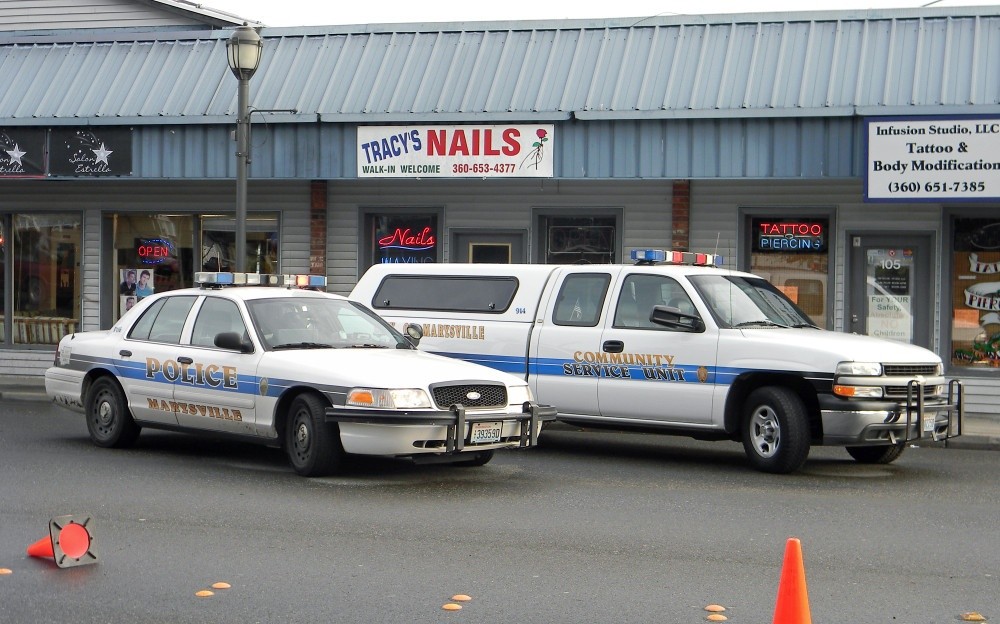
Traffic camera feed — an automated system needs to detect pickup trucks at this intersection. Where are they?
[334,247,968,475]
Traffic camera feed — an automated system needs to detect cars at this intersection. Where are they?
[44,272,558,480]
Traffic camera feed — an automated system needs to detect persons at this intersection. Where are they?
[122,296,134,317]
[137,270,153,295]
[120,270,137,296]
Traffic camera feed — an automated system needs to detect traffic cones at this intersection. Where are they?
[27,511,98,568]
[768,537,814,624]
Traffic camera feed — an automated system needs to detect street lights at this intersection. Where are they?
[221,22,268,275]
